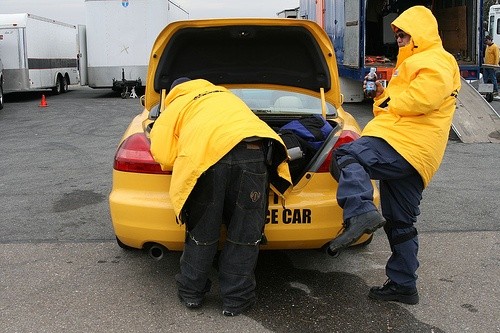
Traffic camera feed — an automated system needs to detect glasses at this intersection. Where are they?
[394,33,408,40]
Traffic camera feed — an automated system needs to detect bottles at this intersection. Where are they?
[364,67,377,99]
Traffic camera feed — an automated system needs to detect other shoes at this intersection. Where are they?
[222,309,236,316]
[182,293,199,307]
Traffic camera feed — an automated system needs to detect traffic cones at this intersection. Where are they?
[38,95,49,107]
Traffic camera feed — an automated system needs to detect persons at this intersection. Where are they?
[150,78,292,316]
[483,36,500,97]
[329,6,462,306]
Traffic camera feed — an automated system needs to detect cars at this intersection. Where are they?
[108,18,381,261]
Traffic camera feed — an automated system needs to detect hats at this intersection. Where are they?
[485,35,492,40]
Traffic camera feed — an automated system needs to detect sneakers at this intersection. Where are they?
[330,210,386,251]
[369,277,419,305]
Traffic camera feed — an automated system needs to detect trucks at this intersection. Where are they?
[0,13,79,95]
[277,0,481,103]
